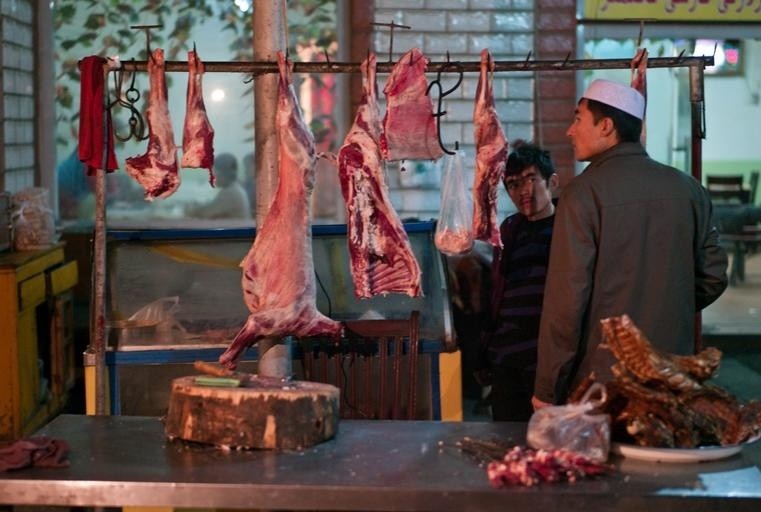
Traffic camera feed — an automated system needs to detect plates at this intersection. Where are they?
[605,432,752,463]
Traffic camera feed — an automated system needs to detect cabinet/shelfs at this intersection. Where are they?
[2,239,80,445]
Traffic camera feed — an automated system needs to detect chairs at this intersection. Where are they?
[299,308,421,420]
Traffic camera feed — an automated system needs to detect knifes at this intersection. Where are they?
[193,376,264,389]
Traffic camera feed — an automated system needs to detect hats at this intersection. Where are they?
[579,78,646,122]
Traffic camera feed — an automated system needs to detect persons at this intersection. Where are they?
[237,152,256,221]
[529,75,731,417]
[457,137,563,423]
[186,151,251,221]
[56,114,136,219]
